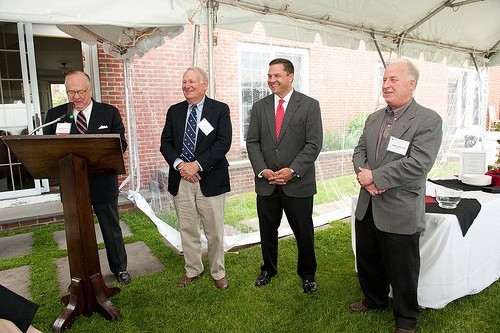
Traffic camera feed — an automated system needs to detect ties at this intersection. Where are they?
[275,100,284,143]
[182,104,197,162]
[75,110,87,135]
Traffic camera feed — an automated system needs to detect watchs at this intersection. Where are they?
[290,168,297,177]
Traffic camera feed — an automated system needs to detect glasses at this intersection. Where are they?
[67,85,90,95]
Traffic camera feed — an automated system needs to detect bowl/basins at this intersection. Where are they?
[435,186,463,209]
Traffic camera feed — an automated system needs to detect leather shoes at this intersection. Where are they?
[396,328,414,333]
[302,279,317,296]
[177,270,204,288]
[349,300,386,312]
[115,271,132,285]
[214,277,227,290]
[255,269,271,286]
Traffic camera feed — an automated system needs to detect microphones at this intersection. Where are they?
[28,115,66,134]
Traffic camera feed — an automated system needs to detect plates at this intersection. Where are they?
[461,174,492,187]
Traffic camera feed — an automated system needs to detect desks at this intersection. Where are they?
[351,176,500,309]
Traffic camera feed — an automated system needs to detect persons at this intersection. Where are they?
[246,58,323,295]
[348,60,443,333]
[43,70,131,294]
[160,67,232,289]
